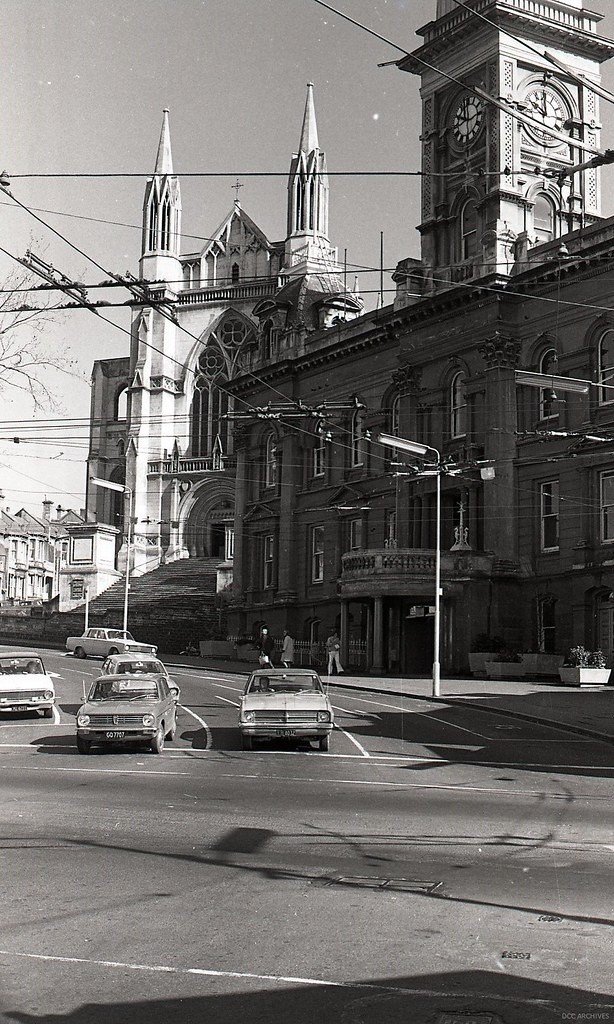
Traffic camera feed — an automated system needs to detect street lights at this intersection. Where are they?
[89,475,132,641]
[376,432,441,697]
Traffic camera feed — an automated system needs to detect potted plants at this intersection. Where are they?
[199,625,235,658]
[484,649,526,680]
[558,646,612,688]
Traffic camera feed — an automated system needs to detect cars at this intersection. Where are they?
[74,674,177,754]
[65,628,159,658]
[239,668,334,752]
[98,653,182,704]
[0,651,57,717]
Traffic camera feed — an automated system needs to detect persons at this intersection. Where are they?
[326,631,345,676]
[281,630,295,669]
[256,628,275,669]
[256,677,275,694]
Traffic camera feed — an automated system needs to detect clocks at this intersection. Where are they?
[443,85,487,153]
[520,81,573,148]
